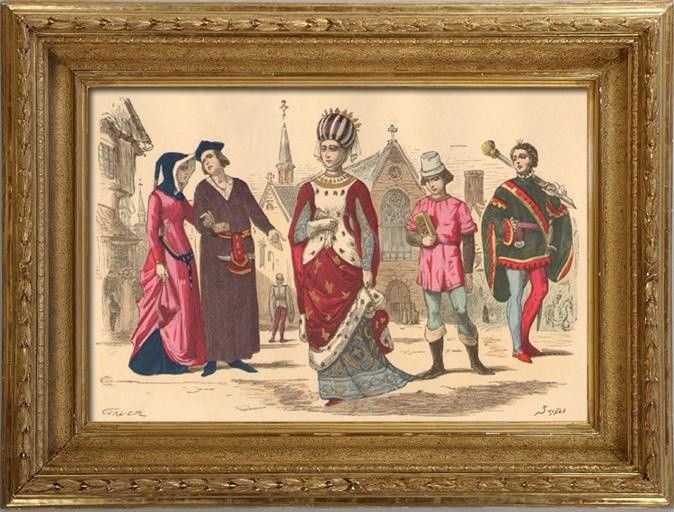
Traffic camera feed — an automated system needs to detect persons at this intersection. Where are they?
[107,294,120,332]
[126,152,214,377]
[403,150,494,379]
[481,138,575,363]
[193,140,286,376]
[287,108,417,406]
[266,272,294,341]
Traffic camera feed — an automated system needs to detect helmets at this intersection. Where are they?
[275,273,284,285]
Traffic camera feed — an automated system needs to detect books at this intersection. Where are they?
[413,210,434,237]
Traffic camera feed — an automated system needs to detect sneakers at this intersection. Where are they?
[523,344,542,357]
[269,339,288,343]
[228,360,258,373]
[200,361,217,377]
[512,350,532,364]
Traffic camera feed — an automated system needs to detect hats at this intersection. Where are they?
[155,152,196,198]
[195,140,224,161]
[316,113,357,151]
[420,150,446,178]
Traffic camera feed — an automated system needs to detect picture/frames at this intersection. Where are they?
[0,0,673,509]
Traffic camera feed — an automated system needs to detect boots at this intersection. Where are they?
[421,324,447,380]
[458,326,490,374]
